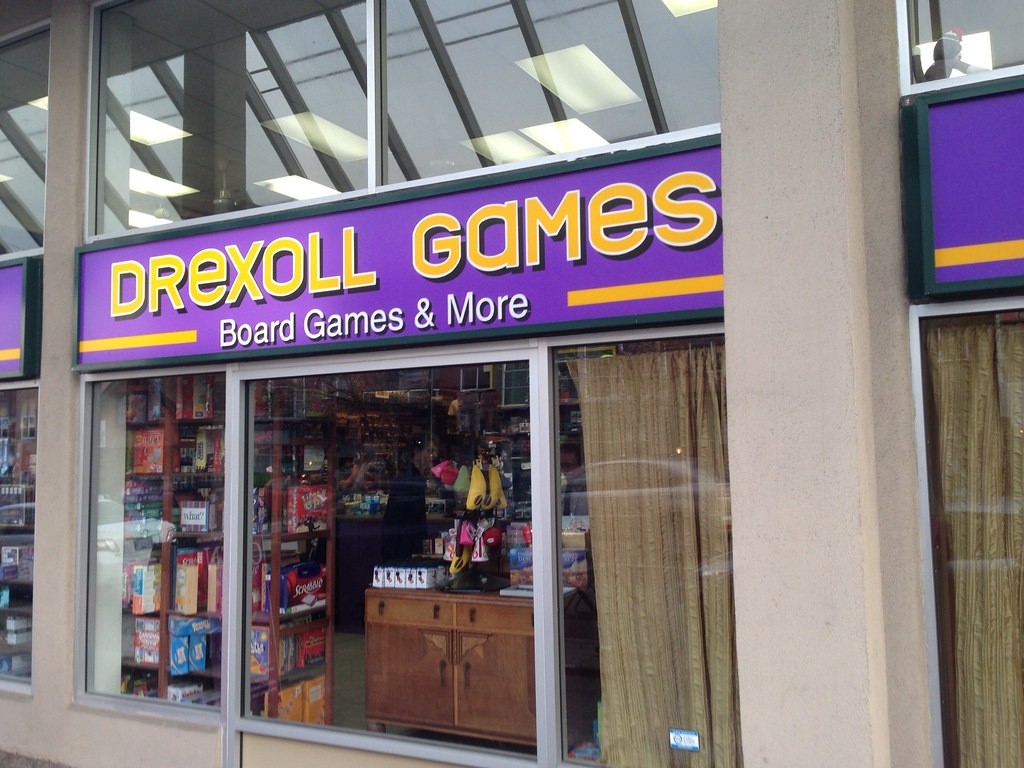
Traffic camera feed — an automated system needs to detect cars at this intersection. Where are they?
[518,458,732,530]
[0,502,177,595]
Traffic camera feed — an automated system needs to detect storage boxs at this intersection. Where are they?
[0,376,591,725]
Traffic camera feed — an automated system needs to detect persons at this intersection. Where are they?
[383,432,440,567]
[560,442,587,515]
[335,462,357,488]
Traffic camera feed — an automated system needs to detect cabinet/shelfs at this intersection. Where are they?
[0,373,340,726]
[364,588,583,746]
